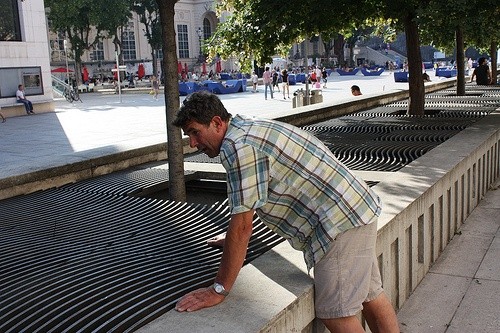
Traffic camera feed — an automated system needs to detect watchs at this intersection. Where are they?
[214,282,229,296]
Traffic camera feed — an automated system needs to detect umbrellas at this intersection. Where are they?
[83,66,89,82]
[178,62,188,74]
[114,65,117,79]
[202,62,207,74]
[217,60,221,73]
[138,64,145,79]
[51,67,74,78]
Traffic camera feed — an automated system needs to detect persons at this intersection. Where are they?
[468,57,492,85]
[124,72,133,84]
[403,61,407,71]
[450,59,454,67]
[16,85,34,114]
[385,61,393,71]
[289,65,305,73]
[351,85,362,96]
[310,66,327,88]
[431,56,434,64]
[181,69,221,80]
[251,67,290,100]
[172,90,401,333]
[384,46,388,53]
[151,75,159,101]
[90,74,102,85]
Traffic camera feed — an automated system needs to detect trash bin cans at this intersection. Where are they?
[311,90,323,104]
[293,89,310,108]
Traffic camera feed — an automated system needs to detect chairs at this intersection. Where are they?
[277,73,305,85]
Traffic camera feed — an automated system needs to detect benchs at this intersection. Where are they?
[394,72,409,82]
[425,62,434,68]
[436,67,458,77]
[179,79,242,96]
[337,64,385,76]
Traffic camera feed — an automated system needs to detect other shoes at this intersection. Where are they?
[27,111,35,115]
